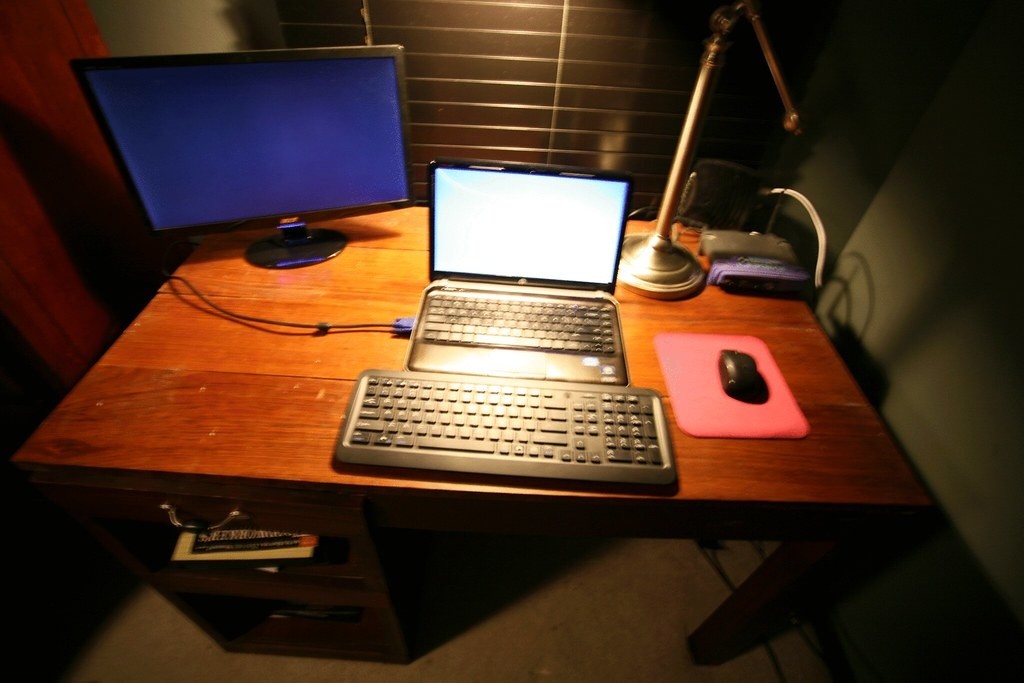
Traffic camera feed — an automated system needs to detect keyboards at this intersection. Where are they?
[332,371,678,495]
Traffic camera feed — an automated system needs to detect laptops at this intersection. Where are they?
[404,157,636,396]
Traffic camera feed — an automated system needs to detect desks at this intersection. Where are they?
[8,205,938,665]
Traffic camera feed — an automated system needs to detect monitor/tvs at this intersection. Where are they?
[70,44,416,268]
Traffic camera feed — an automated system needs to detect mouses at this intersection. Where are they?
[717,350,761,402]
